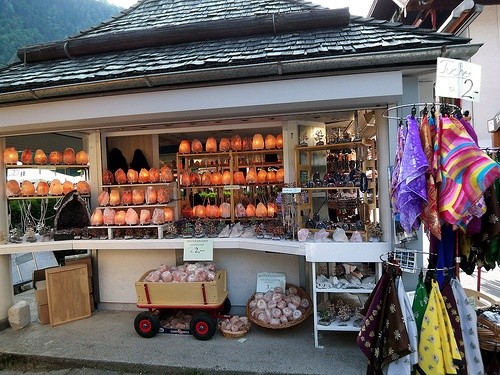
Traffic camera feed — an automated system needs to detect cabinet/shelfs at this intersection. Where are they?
[176,149,285,223]
[311,262,382,348]
[88,181,178,239]
[293,139,377,242]
[5,164,90,200]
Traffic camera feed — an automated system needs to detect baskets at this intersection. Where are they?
[222,329,247,339]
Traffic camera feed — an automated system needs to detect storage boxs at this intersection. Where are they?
[31,258,95,325]
[134,269,226,305]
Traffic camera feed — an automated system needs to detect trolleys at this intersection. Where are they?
[134,269,231,341]
